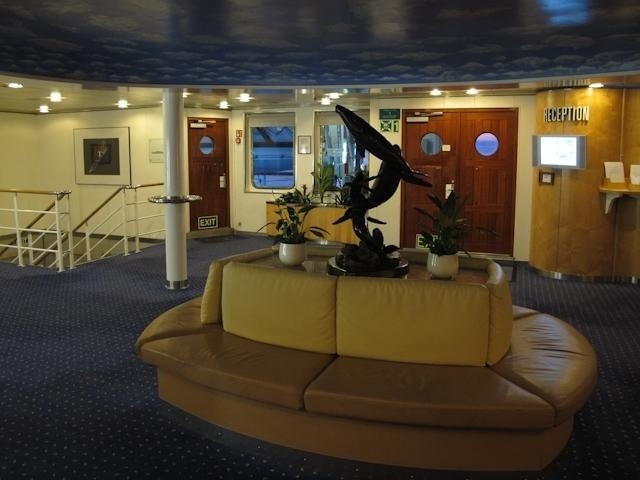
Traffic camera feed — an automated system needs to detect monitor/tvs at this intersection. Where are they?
[531,135,587,173]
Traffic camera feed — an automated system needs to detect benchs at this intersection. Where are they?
[134,244,598,472]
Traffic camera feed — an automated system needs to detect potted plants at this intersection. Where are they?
[411,190,503,278]
[256,184,331,266]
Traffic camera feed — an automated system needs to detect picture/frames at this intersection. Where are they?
[73,126,131,186]
[298,136,311,154]
[539,171,554,185]
